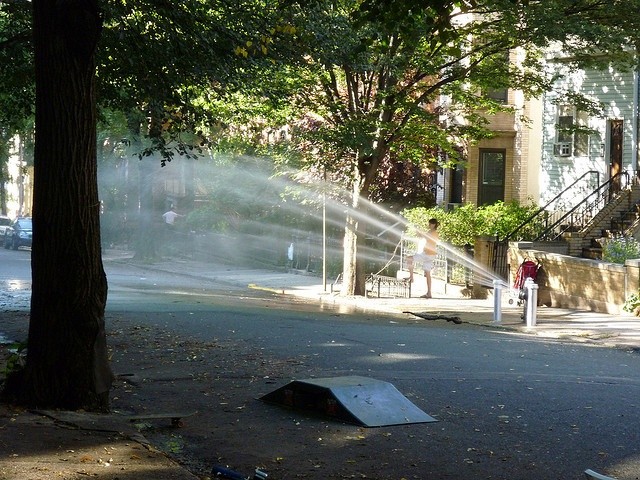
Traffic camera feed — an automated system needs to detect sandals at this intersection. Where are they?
[404,277,413,282]
[420,294,433,298]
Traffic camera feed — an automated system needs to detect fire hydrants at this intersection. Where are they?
[518,277,539,327]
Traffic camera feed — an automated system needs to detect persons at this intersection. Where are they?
[161,205,187,229]
[406,218,440,298]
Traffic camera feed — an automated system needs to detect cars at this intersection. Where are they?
[5,215,33,251]
[0,215,12,246]
